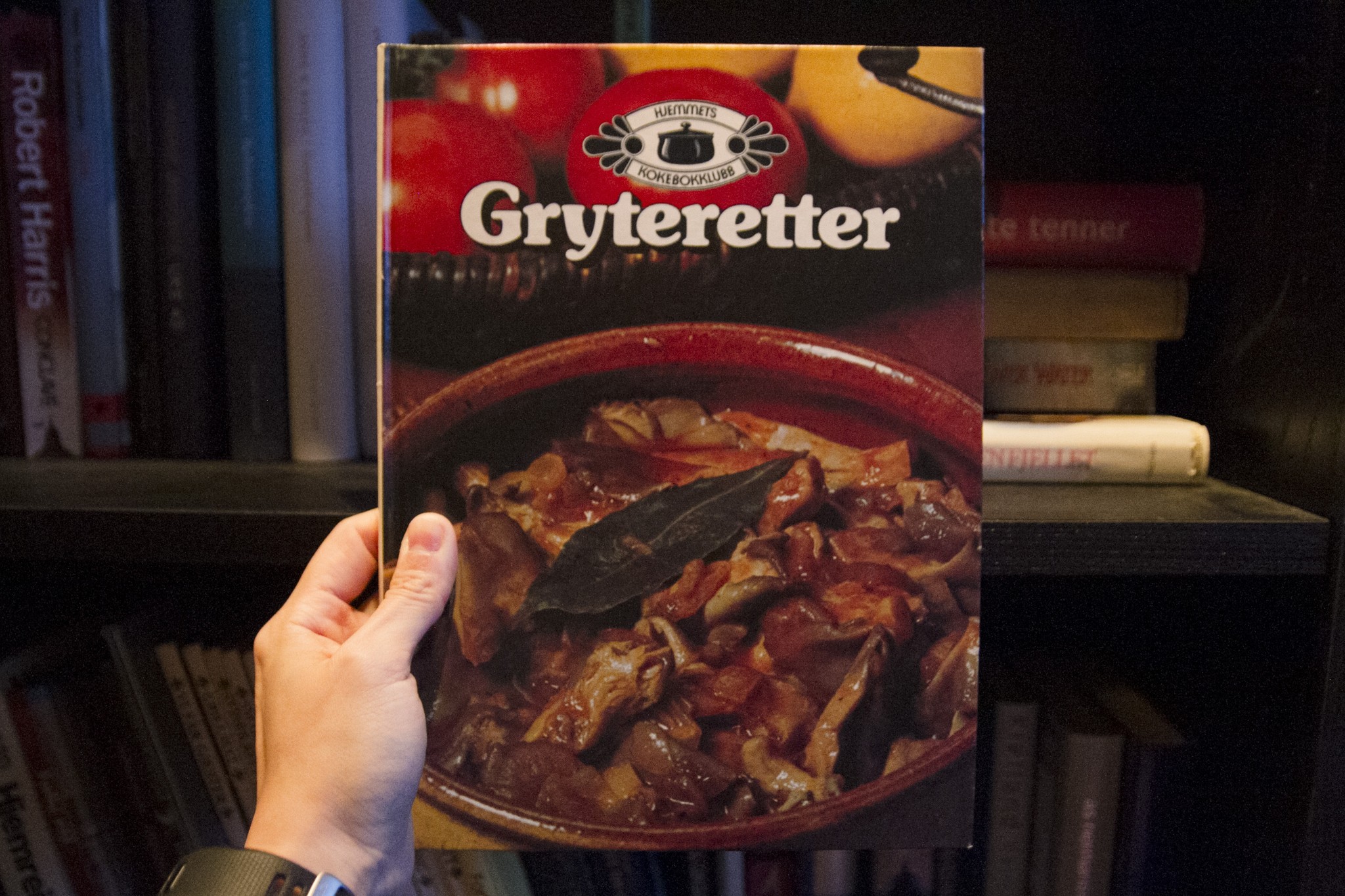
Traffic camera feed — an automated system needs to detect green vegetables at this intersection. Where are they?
[509,451,807,630]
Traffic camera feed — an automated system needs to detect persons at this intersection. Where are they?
[157,508,459,896]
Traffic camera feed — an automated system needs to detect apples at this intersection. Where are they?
[380,50,811,256]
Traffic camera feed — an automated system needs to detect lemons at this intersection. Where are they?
[587,48,986,176]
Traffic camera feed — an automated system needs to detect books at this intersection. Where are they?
[379,43,981,853]
[0,0,1219,896]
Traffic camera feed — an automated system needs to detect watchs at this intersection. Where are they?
[159,847,356,896]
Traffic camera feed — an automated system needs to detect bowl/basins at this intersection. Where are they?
[379,324,977,856]
